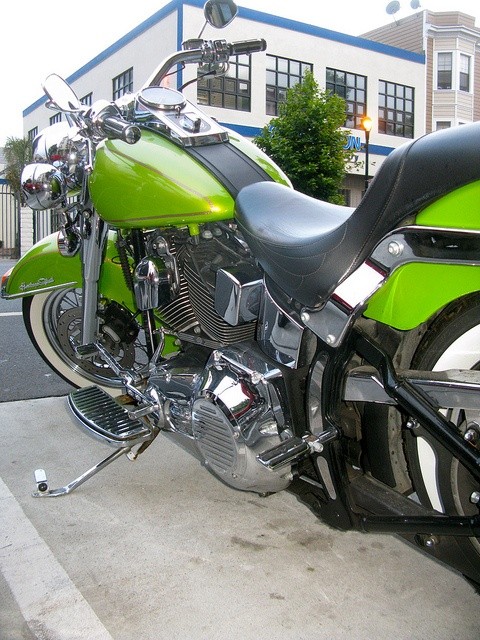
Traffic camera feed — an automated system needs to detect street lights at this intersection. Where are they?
[363,119,373,194]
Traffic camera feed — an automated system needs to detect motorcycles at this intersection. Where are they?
[0,0,480,594]
